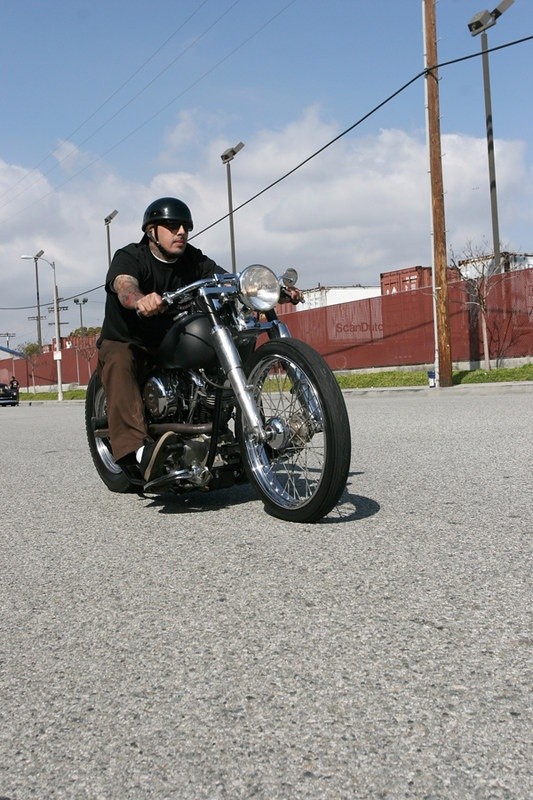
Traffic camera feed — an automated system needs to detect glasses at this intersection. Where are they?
[155,219,193,232]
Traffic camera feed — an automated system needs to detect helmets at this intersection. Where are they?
[141,197,194,231]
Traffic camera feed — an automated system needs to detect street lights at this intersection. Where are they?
[73,296,88,329]
[21,256,63,402]
[104,209,118,265]
[466,0,518,274]
[35,250,47,348]
[221,141,245,274]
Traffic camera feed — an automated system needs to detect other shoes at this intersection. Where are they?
[264,443,280,460]
[138,431,176,483]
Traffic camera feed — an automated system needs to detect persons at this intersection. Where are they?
[10,376,20,406]
[95,198,305,484]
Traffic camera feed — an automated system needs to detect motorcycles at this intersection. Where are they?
[83,265,354,524]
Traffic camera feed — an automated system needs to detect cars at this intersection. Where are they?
[0,382,19,407]
